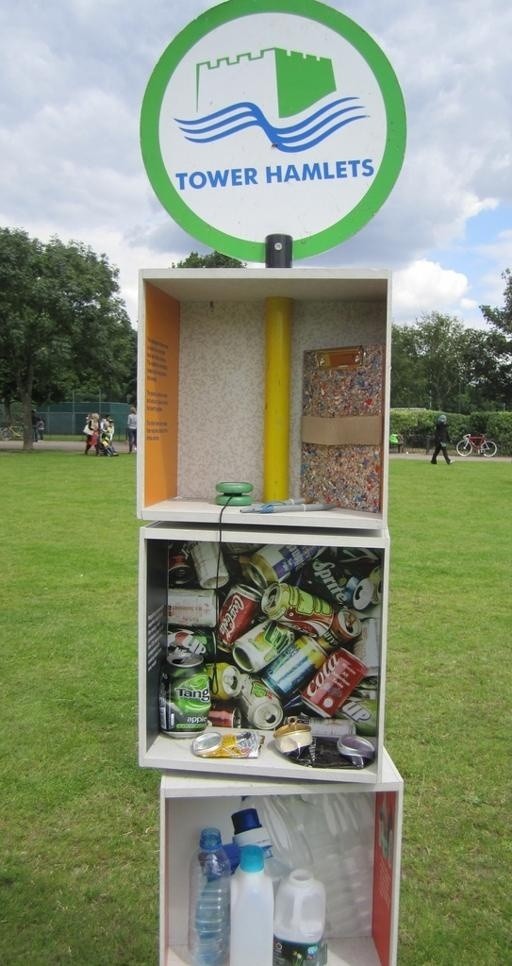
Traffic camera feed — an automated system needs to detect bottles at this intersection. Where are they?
[184,827,231,966]
[227,846,272,966]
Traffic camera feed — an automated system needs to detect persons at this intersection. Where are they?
[37,414,44,440]
[83,412,119,456]
[126,406,137,453]
[31,407,38,442]
[430,414,456,464]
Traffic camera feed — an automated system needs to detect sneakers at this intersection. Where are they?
[449,460,455,464]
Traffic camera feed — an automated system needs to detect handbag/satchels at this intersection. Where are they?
[83,425,95,435]
[440,442,446,447]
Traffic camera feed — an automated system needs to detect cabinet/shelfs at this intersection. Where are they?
[136,267,404,966]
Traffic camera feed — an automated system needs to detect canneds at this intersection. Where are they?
[272,724,375,768]
[191,723,264,758]
[159,543,383,736]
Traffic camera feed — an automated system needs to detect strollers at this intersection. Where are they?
[99,429,114,456]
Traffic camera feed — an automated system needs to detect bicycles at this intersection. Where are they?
[456,434,497,457]
[1,424,23,441]
